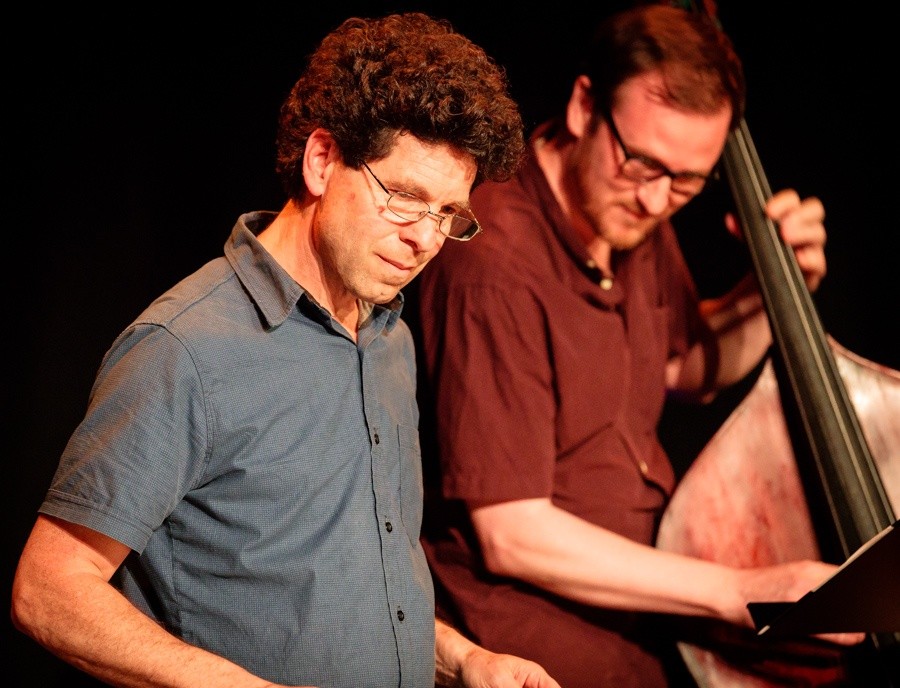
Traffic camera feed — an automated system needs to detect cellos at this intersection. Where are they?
[655,118,900,688]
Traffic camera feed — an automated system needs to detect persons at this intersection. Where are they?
[412,0,866,688]
[13,13,561,688]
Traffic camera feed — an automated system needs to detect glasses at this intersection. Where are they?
[595,87,720,197]
[360,159,484,241]
